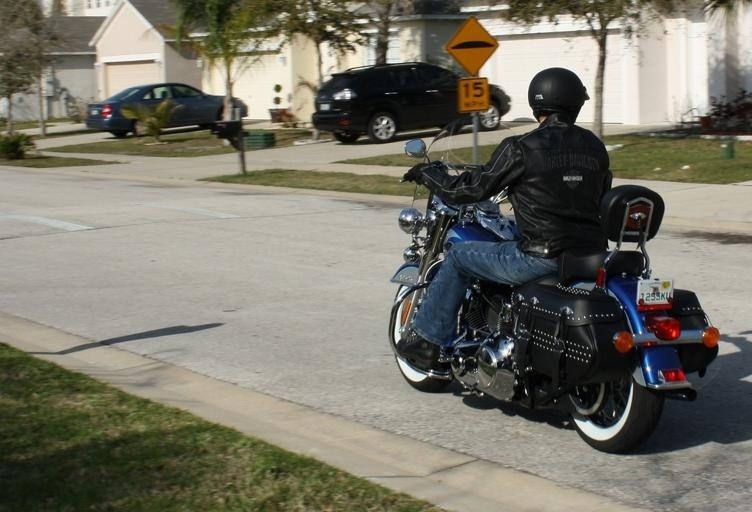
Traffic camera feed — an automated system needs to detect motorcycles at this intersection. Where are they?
[378,133,721,459]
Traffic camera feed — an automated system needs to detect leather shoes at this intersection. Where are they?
[396,336,440,369]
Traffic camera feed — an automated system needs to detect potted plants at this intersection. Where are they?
[269,83,289,122]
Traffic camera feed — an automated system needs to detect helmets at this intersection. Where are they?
[529,69,589,116]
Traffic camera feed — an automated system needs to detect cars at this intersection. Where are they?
[82,80,251,140]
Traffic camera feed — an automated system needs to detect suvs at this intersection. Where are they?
[310,59,513,145]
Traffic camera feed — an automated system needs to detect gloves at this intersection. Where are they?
[408,163,428,185]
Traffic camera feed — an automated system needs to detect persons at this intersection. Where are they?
[396,67,614,369]
